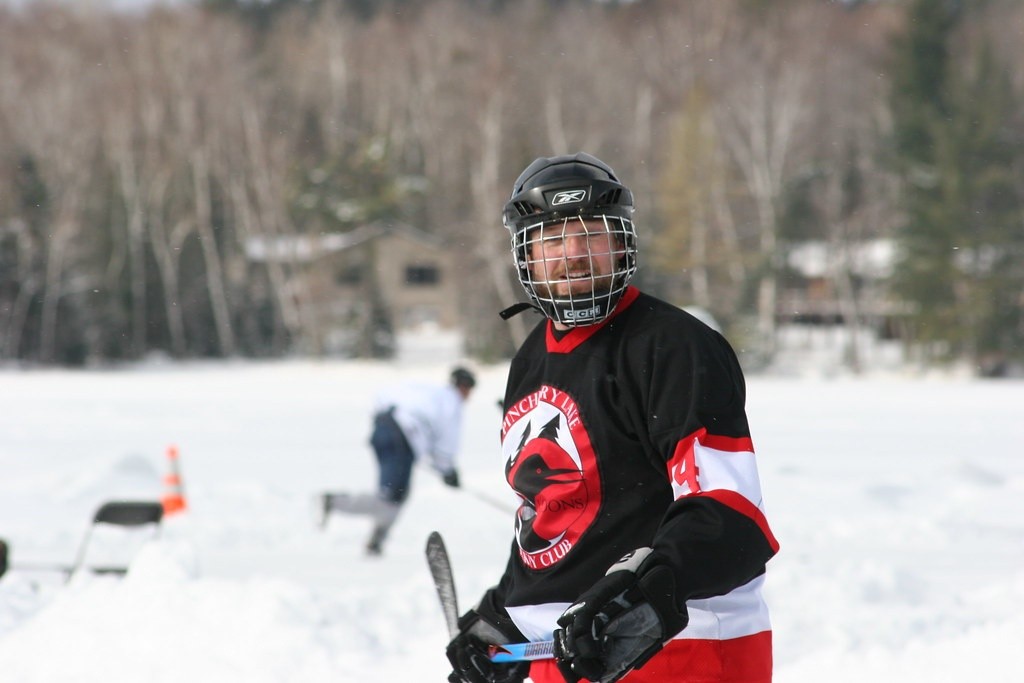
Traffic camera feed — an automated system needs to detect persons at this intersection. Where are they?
[447,154,780,683]
[318,367,475,558]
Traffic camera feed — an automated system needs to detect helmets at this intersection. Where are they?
[502,151,638,328]
[452,369,476,389]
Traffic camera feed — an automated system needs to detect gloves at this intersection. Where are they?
[446,588,534,683]
[552,546,689,683]
[446,472,458,487]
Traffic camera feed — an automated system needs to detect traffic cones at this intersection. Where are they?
[156,447,187,520]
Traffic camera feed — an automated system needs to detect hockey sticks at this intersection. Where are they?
[425,530,556,663]
